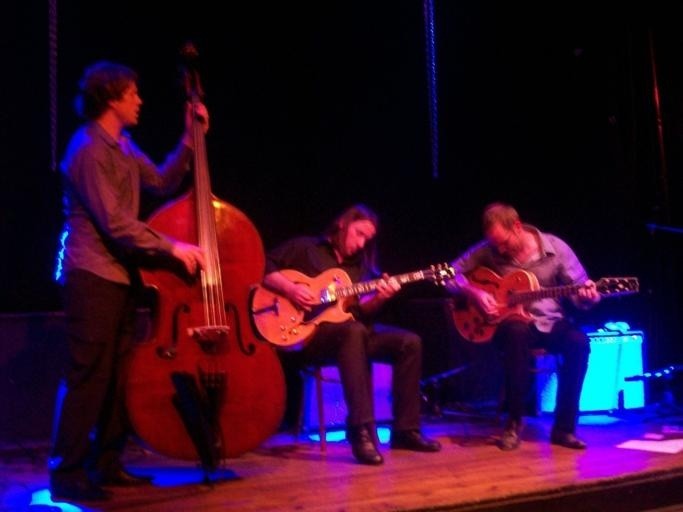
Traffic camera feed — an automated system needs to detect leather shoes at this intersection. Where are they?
[502,418,519,450]
[390,430,441,450]
[553,428,586,448]
[352,425,382,463]
[50,470,112,501]
[98,468,147,487]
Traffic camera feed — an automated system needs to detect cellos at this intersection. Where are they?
[126,41,285,458]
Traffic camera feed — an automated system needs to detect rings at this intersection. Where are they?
[189,259,196,265]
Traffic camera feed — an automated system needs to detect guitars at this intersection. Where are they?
[447,265,640,343]
[252,261,456,347]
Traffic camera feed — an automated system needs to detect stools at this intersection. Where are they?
[490,346,548,438]
[292,354,372,450]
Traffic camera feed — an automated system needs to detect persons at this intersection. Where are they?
[443,202,601,453]
[46,59,209,502]
[259,202,440,462]
